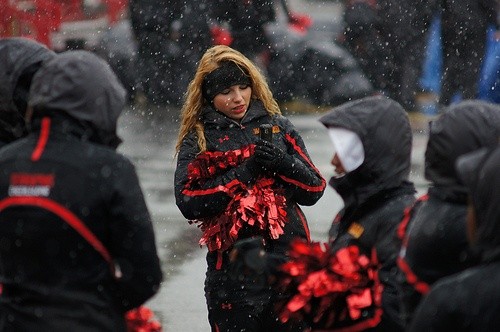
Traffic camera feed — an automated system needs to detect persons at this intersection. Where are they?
[0,34,59,150]
[0,48,163,331]
[396,100,500,332]
[409,148,500,332]
[317,94,419,331]
[173,44,326,332]
[123,1,500,121]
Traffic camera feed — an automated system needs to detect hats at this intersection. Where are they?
[329,124,365,171]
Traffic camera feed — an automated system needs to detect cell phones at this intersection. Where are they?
[260,124,273,144]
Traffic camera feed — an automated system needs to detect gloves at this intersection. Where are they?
[254,140,294,175]
[245,153,267,177]
